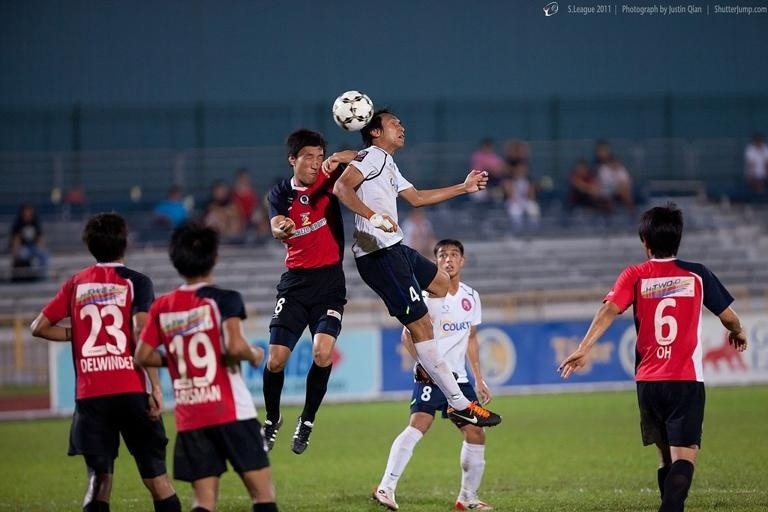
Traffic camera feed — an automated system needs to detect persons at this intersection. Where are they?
[29,211,184,512]
[8,203,50,282]
[743,133,767,198]
[401,201,440,263]
[256,126,360,455]
[142,182,194,249]
[466,133,648,229]
[556,205,749,511]
[133,220,282,511]
[332,104,502,426]
[203,181,245,246]
[231,165,267,236]
[375,238,501,511]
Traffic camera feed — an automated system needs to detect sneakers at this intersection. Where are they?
[291,416,314,454]
[455,500,494,511]
[447,400,501,429]
[260,412,283,452]
[373,487,399,511]
[415,363,458,384]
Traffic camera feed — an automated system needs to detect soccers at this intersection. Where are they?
[332,91,375,131]
